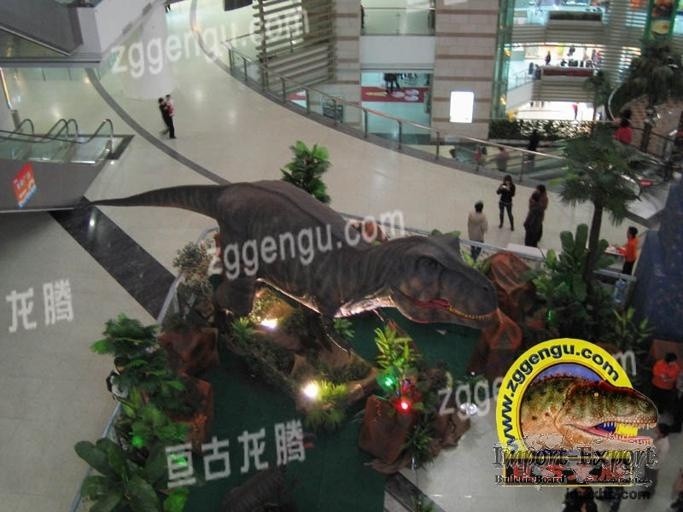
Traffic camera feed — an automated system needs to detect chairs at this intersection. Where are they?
[635,177,656,201]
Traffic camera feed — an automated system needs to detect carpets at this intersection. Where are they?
[360,87,429,102]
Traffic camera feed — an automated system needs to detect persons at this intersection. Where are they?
[650,349,680,418]
[467,201,488,264]
[155,94,176,140]
[545,47,600,67]
[523,185,549,248]
[497,174,515,232]
[495,140,512,170]
[616,226,638,280]
[526,127,541,161]
[382,76,403,95]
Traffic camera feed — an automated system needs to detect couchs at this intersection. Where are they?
[535,63,595,81]
